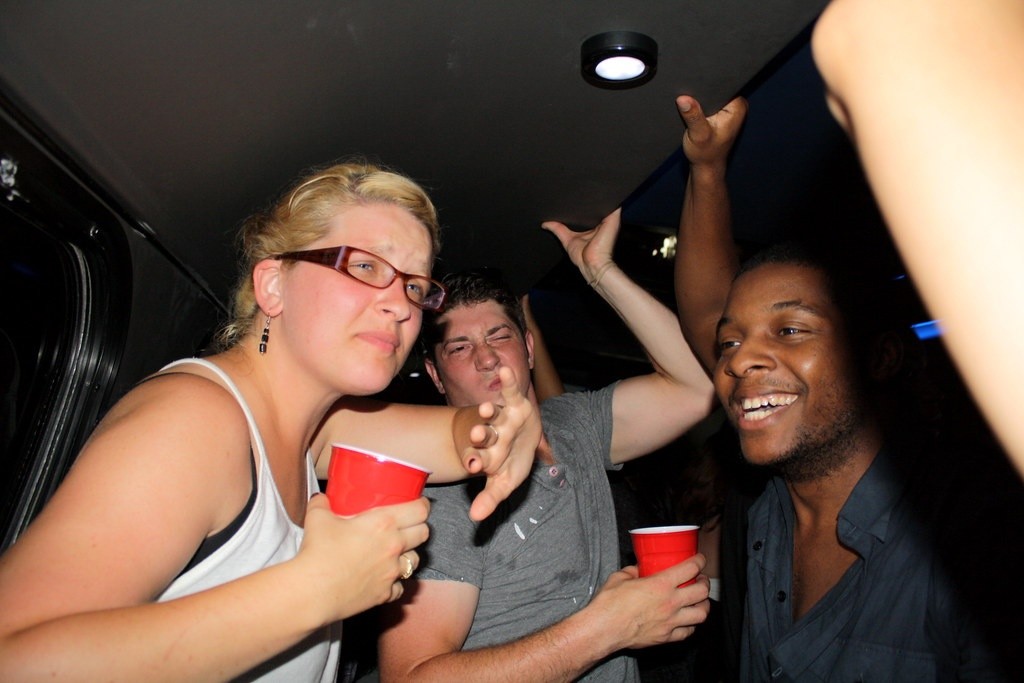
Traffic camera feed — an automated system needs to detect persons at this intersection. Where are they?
[811,0,1024,478]
[672,91,1024,683]
[378,207,715,683]
[0,161,543,683]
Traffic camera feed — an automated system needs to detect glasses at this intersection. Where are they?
[267,246,449,313]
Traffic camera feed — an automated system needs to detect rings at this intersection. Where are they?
[386,582,401,602]
[399,554,413,580]
[485,425,498,448]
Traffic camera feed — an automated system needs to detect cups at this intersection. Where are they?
[628,525,701,589]
[325,442,433,519]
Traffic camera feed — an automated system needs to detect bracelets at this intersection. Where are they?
[588,261,617,289]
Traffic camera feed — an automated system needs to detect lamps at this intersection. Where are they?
[577,31,659,91]
